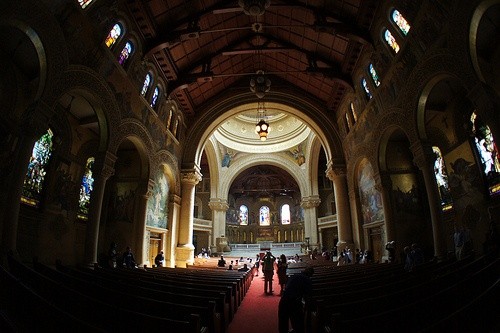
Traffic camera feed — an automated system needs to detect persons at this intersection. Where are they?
[155,250,164,267]
[278,267,319,333]
[295,238,422,268]
[198,247,209,258]
[276,254,289,294]
[218,255,226,267]
[453,225,464,255]
[227,256,252,271]
[262,252,276,294]
[393,139,500,199]
[254,254,260,276]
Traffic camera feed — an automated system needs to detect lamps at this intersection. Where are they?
[256,95,270,142]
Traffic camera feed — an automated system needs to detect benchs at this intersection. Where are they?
[0,256,257,333]
[296,252,500,333]
